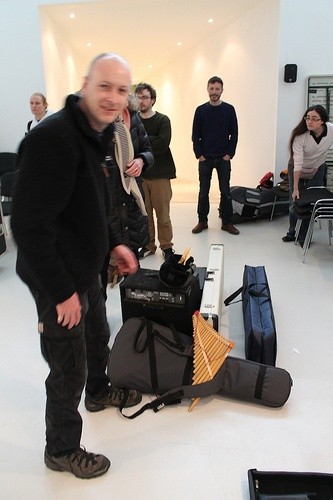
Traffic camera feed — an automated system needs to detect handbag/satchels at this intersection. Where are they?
[224,265,277,368]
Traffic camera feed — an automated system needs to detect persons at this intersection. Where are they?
[25,92,54,136]
[111,92,154,279]
[191,76,239,235]
[134,82,176,260]
[10,52,142,478]
[281,104,333,241]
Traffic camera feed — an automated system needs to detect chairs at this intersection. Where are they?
[0,152,18,256]
[270,160,333,263]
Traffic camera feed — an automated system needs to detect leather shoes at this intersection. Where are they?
[221,223,239,235]
[192,223,208,233]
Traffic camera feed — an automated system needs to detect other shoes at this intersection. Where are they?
[282,234,296,242]
[297,236,305,248]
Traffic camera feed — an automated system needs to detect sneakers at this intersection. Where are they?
[85,384,142,412]
[43,445,111,479]
[138,246,155,260]
[162,249,174,259]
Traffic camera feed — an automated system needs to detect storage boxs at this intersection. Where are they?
[199,244,225,333]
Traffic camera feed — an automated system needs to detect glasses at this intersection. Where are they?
[136,96,151,99]
[304,116,322,122]
